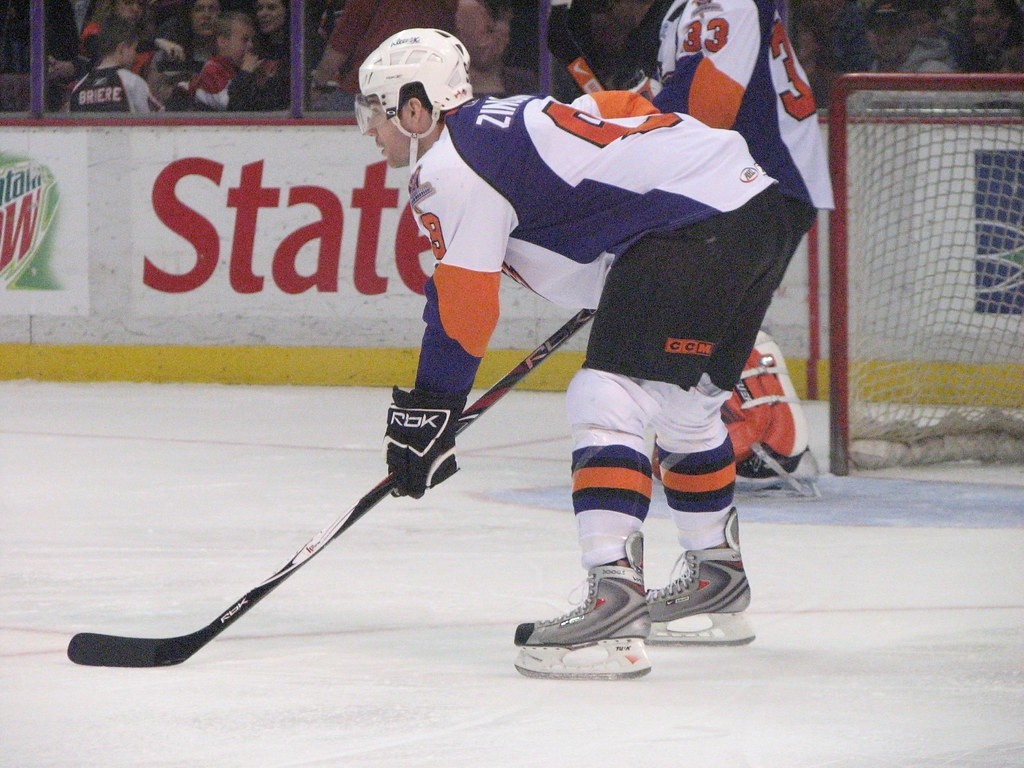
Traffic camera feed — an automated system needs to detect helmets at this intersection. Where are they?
[358,28,473,120]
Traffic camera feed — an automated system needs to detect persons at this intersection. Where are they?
[793,0,1024,109]
[0,0,670,116]
[572,0,834,492]
[353,29,818,646]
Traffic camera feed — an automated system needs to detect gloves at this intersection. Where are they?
[382,386,467,500]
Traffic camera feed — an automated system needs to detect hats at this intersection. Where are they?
[863,1,907,28]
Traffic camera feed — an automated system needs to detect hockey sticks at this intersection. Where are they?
[544,0,606,96]
[66,308,599,670]
[720,402,820,497]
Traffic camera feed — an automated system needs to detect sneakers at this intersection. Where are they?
[514,530,650,681]
[642,507,755,648]
